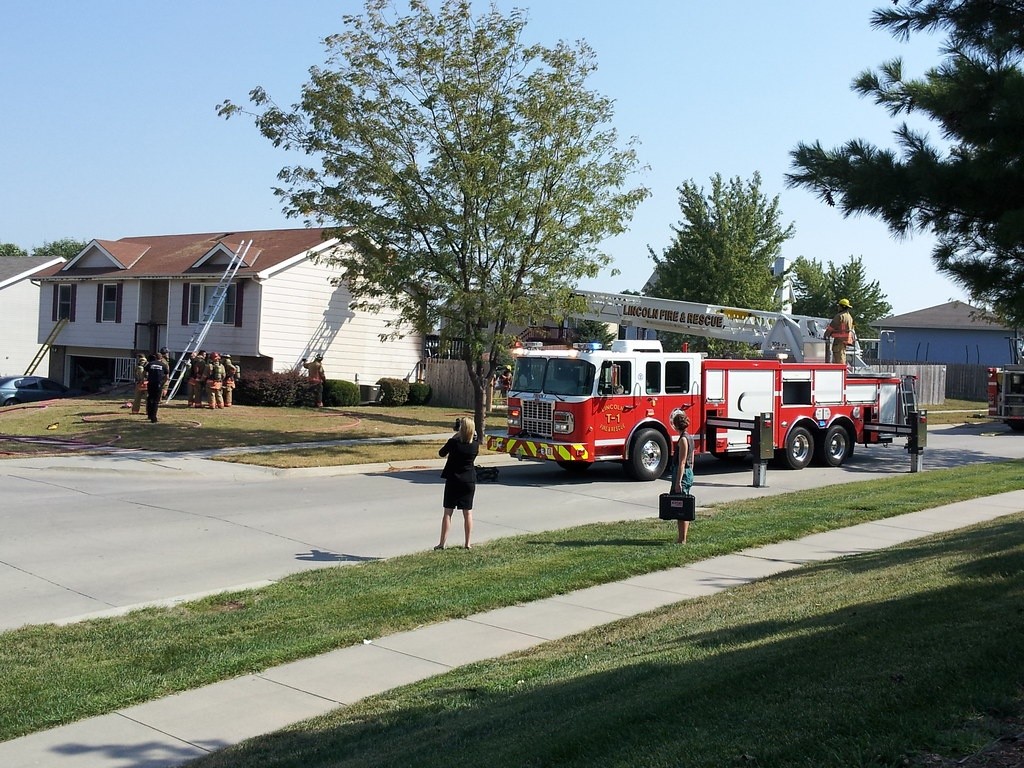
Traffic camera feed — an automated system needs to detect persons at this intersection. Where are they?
[303,354,326,408]
[668,414,693,544]
[131,348,236,423]
[824,299,853,364]
[434,417,479,550]
[492,365,512,405]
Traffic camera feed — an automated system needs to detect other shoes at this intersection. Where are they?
[434,546,445,550]
[464,546,472,549]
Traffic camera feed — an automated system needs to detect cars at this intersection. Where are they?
[0,377,92,406]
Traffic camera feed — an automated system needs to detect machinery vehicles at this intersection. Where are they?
[488,275,927,489]
[987,353,1024,432]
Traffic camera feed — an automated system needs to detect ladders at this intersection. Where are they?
[23,318,69,376]
[160,238,254,404]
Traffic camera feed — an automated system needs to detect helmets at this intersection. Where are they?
[139,357,148,362]
[210,351,218,360]
[159,347,170,353]
[839,298,852,308]
[315,353,323,359]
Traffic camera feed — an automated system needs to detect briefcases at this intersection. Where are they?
[659,489,695,521]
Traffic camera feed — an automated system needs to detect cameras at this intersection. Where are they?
[453,419,461,431]
